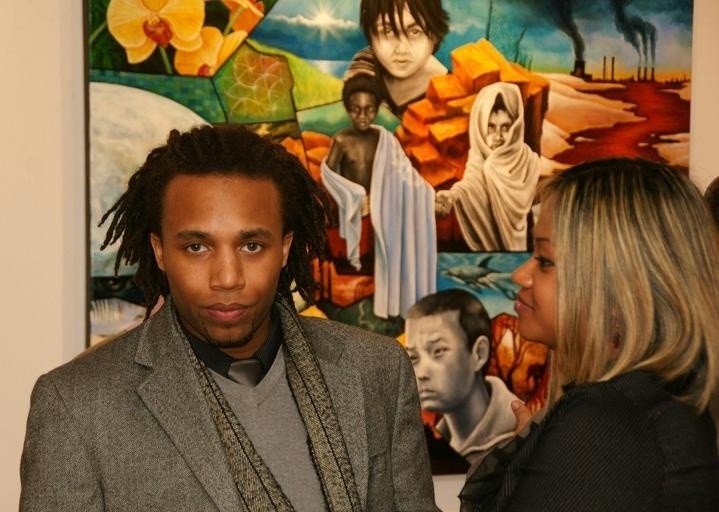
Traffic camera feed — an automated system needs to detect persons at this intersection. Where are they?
[360,0,453,118]
[318,46,446,323]
[703,178,719,222]
[459,157,719,512]
[404,288,526,473]
[437,81,571,252]
[18,122,445,512]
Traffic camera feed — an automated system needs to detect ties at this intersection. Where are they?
[228,358,263,389]
